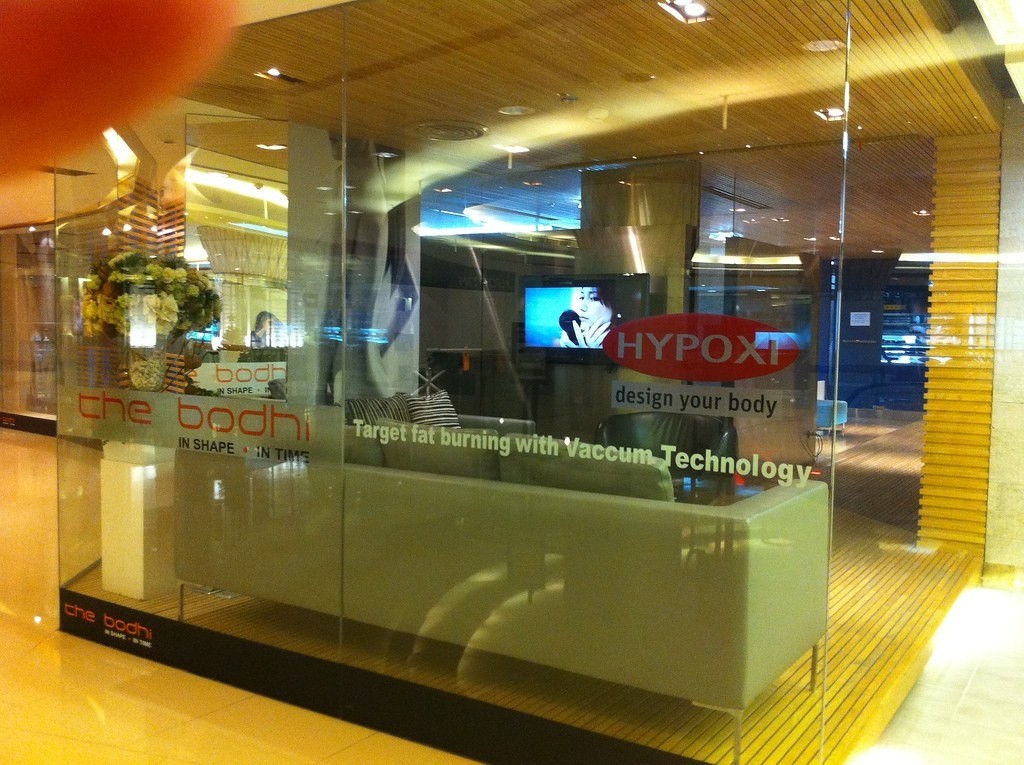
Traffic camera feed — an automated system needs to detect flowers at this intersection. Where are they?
[79,250,224,337]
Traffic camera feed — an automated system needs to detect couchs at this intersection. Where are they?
[173,414,835,765]
[595,410,738,484]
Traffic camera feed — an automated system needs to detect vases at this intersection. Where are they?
[128,334,167,392]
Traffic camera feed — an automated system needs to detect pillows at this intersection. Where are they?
[497,432,676,502]
[338,393,411,426]
[260,422,385,467]
[398,390,461,429]
[375,418,500,481]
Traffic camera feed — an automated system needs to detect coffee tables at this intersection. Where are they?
[672,472,777,506]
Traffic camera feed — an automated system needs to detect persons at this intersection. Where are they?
[250,311,282,350]
[559,287,615,349]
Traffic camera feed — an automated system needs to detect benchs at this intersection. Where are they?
[817,399,848,436]
